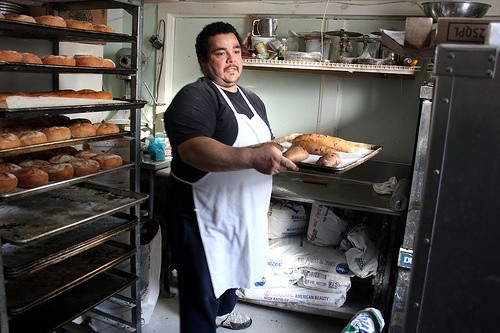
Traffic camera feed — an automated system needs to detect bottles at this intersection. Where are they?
[250,37,275,50]
[278,38,288,60]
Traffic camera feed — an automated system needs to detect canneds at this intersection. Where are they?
[255,42,269,56]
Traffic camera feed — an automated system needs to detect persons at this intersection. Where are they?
[161,22,298,333]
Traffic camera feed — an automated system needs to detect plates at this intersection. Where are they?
[0,1,23,14]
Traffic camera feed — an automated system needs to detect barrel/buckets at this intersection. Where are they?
[89,141,130,191]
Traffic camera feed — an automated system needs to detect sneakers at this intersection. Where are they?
[214,309,252,329]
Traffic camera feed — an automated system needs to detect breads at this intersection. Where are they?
[0,13,122,194]
[280,133,360,168]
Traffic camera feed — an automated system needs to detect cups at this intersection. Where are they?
[252,18,274,37]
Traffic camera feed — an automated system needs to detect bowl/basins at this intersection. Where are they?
[421,2,492,23]
[306,37,400,64]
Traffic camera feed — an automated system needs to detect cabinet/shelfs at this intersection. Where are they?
[0,0,145,333]
